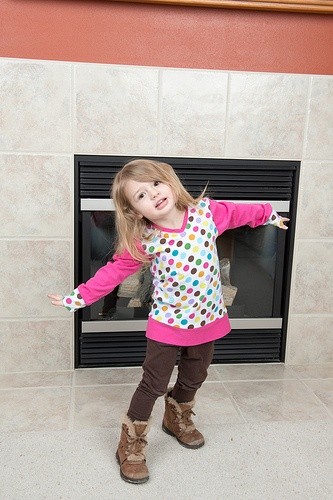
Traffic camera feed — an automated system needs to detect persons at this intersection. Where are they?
[47,159,290,484]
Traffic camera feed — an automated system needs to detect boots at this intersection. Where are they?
[161,388,204,449]
[115,415,150,484]
